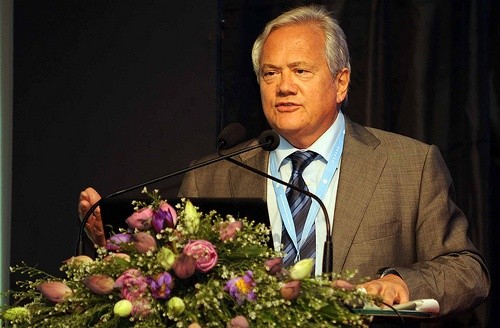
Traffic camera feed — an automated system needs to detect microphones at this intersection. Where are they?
[216,122,333,282]
[75,130,279,256]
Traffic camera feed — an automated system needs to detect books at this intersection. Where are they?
[363,298,440,313]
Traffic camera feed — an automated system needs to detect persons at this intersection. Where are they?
[79,5,490,314]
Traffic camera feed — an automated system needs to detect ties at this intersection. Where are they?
[277,149,320,281]
